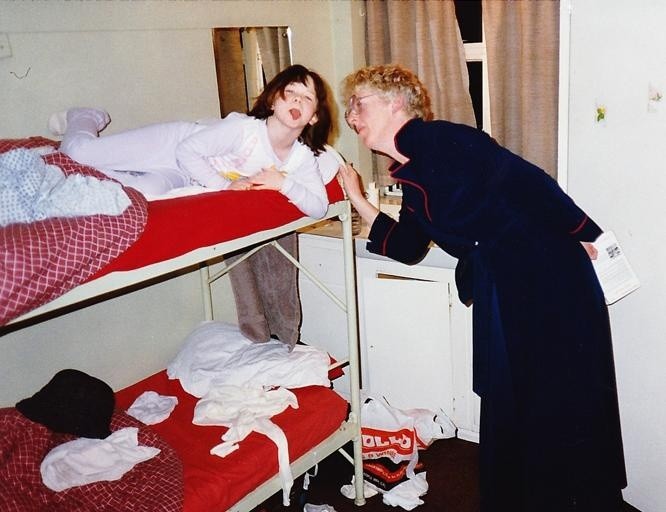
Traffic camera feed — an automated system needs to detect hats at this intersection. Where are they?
[14,369,116,440]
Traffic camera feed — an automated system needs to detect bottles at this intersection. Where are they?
[364,182,380,209]
[352,207,361,236]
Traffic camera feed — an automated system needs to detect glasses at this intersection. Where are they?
[343,92,385,130]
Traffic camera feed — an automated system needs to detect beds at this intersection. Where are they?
[0,138,370,512]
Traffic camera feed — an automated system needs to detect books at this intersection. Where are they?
[588,230,640,306]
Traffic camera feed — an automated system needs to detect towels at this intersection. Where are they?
[38,390,178,494]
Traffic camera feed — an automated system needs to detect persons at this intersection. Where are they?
[49,62,338,223]
[338,64,627,512]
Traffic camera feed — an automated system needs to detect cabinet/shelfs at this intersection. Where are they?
[297,196,479,446]
